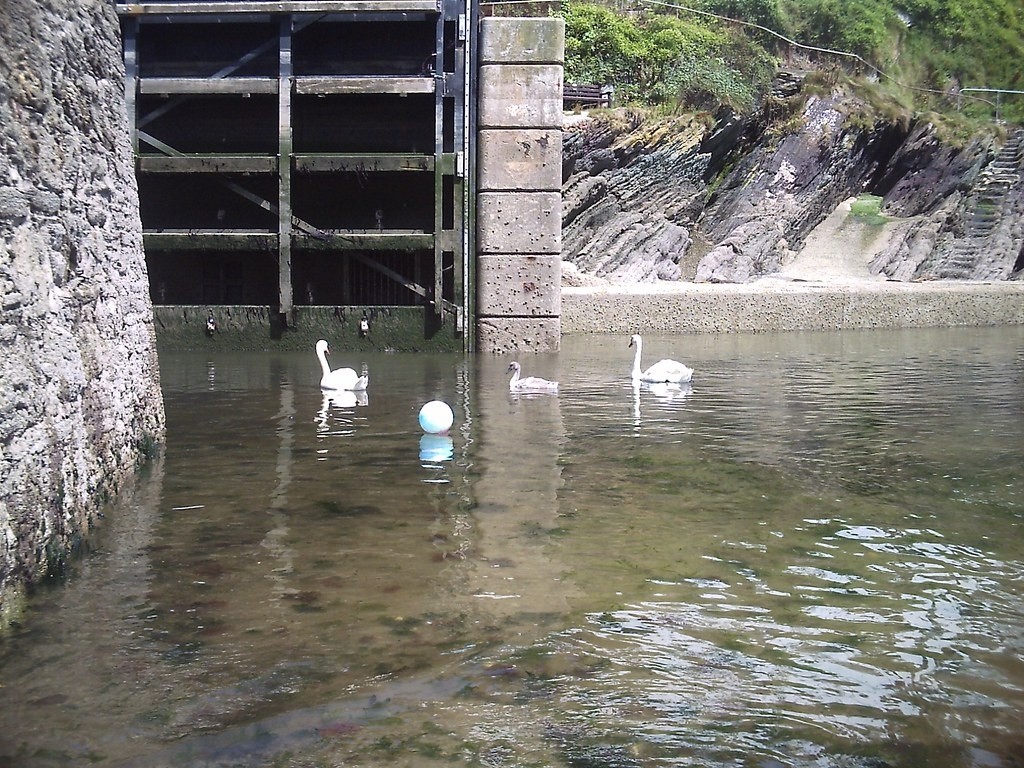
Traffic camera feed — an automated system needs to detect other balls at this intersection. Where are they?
[418,399,454,434]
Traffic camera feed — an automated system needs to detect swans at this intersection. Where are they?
[506,362,558,390]
[628,334,694,382]
[315,339,368,390]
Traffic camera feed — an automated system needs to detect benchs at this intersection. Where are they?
[564,82,612,109]
